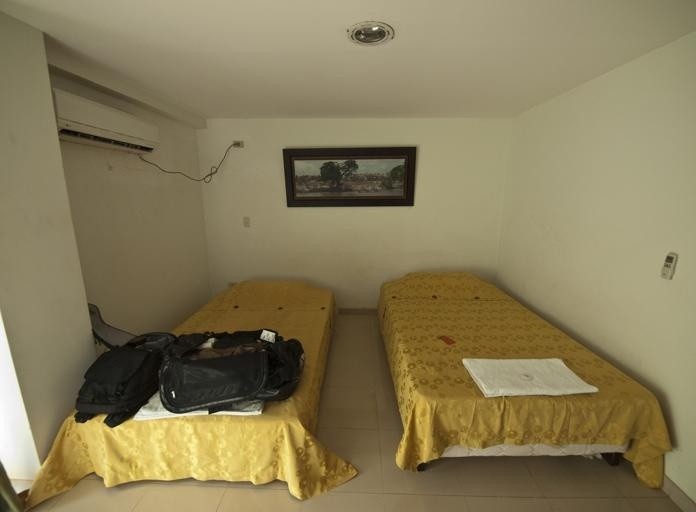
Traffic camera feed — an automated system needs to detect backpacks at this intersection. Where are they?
[75,333,176,429]
[158,329,304,414]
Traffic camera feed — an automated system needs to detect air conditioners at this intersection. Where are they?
[52,88,160,156]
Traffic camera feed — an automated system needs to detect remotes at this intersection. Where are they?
[660,252,677,280]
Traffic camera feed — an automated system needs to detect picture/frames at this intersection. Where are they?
[282,146,418,208]
